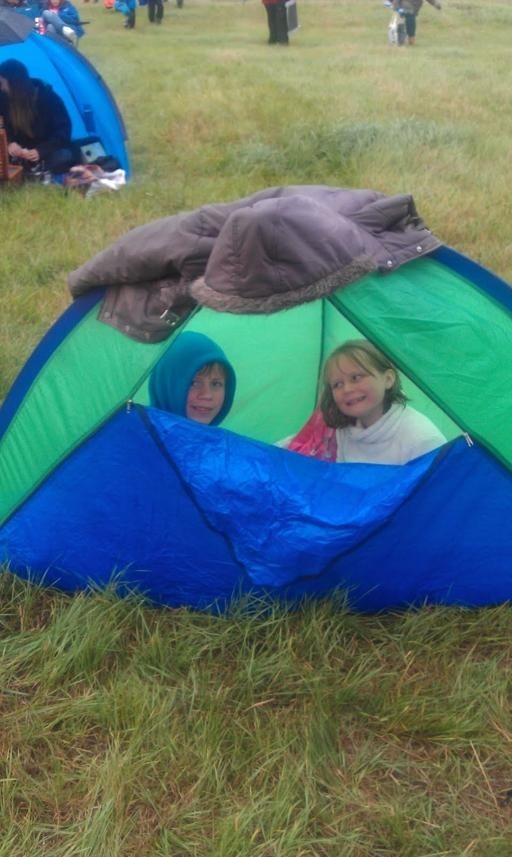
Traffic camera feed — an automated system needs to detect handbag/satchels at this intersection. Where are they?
[388,12,406,47]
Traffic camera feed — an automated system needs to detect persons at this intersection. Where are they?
[115,0,184,28]
[390,0,443,45]
[1,0,85,44]
[0,60,80,184]
[150,331,238,429]
[309,347,448,468]
[261,0,289,45]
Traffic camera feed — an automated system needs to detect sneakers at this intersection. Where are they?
[62,26,74,36]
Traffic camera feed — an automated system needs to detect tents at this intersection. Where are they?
[0,4,132,178]
[1,186,510,617]
